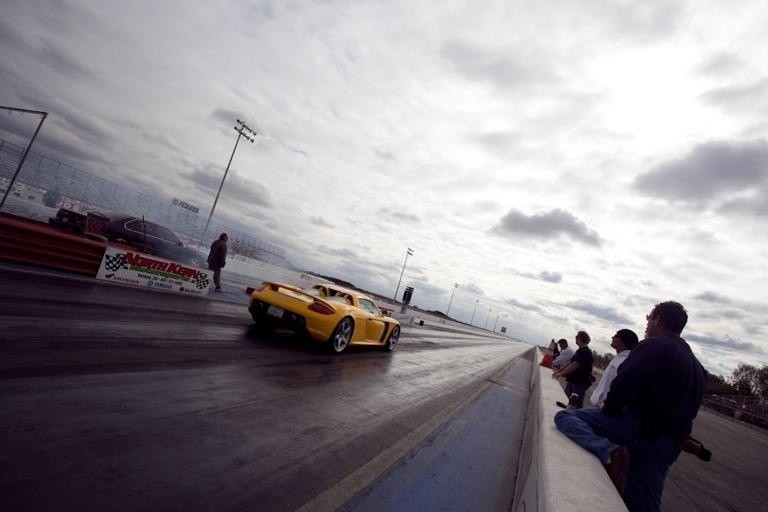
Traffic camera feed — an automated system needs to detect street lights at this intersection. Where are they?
[199,118,256,250]
[493,315,500,332]
[469,299,480,325]
[393,248,414,302]
[484,308,492,328]
[446,283,459,317]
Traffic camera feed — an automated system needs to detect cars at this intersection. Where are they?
[48,208,210,273]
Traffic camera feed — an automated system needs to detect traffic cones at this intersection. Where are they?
[538,339,555,370]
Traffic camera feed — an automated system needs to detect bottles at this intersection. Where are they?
[567,393,579,409]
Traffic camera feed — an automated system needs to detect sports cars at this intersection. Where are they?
[244,280,401,354]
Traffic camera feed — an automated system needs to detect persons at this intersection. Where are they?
[588,329,639,412]
[553,330,596,409]
[549,337,574,370]
[553,300,705,511]
[42,186,62,208]
[206,232,228,290]
